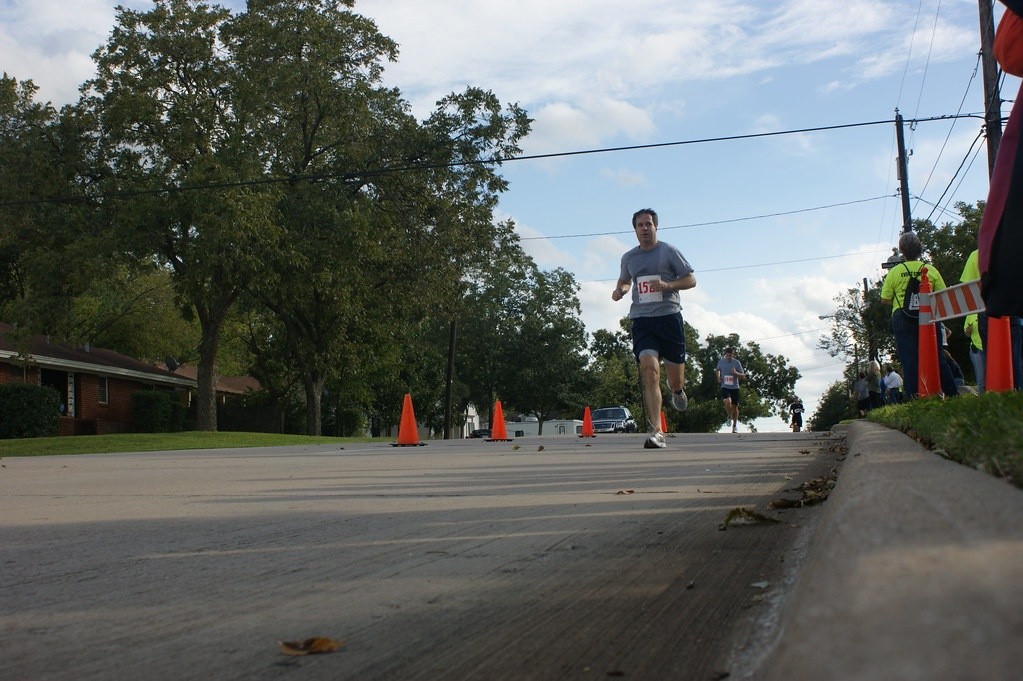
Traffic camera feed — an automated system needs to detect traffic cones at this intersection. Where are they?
[389,394,428,447]
[577,406,597,438]
[983,314,1016,393]
[916,267,943,401]
[482,401,515,442]
[660,409,670,434]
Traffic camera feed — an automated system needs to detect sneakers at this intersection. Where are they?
[644,429,666,447]
[727,416,731,427]
[666,379,687,411]
[732,426,737,433]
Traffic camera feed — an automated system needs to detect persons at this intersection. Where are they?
[789,397,804,432]
[964,314,985,393]
[960,249,1023,391]
[612,208,696,448]
[881,366,904,405]
[993,0,1023,77]
[881,232,960,401]
[716,348,746,433]
[865,361,882,408]
[941,322,964,385]
[855,372,870,417]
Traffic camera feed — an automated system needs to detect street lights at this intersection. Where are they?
[818,315,875,362]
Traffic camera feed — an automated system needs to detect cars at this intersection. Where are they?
[591,405,640,434]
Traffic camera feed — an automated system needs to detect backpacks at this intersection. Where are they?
[894,261,926,317]
